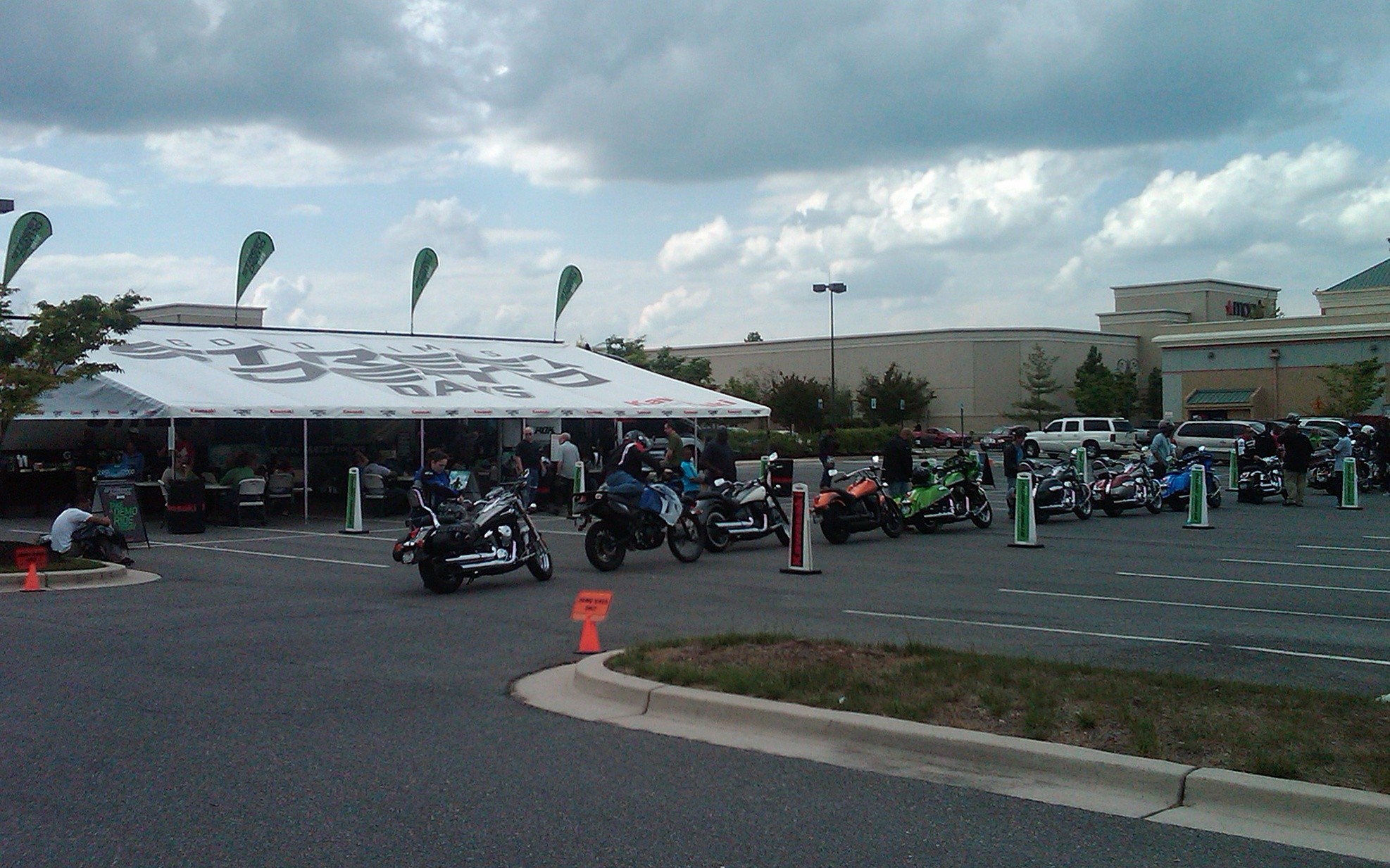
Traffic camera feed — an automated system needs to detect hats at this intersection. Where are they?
[1014,428,1027,437]
[130,426,139,433]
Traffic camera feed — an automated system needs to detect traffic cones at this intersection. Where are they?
[19,564,47,592]
[573,613,607,655]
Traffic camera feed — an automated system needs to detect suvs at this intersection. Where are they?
[1021,417,1137,460]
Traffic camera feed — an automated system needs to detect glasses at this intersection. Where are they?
[1247,430,1253,433]
[526,433,532,435]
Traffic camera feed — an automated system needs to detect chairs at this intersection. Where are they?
[362,474,393,520]
[234,473,293,527]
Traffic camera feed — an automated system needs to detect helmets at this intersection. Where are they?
[623,429,653,451]
[1350,423,1362,429]
[1361,425,1376,436]
[1158,419,1176,432]
[1286,411,1300,422]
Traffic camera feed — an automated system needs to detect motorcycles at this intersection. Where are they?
[1238,449,1289,505]
[893,450,993,534]
[1158,445,1222,512]
[1006,447,1093,524]
[1307,439,1390,496]
[573,472,706,573]
[393,468,554,595]
[812,455,906,545]
[1092,442,1164,518]
[695,452,790,554]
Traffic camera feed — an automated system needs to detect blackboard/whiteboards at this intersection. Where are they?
[92,480,148,543]
[978,452,994,486]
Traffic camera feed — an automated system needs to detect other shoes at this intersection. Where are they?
[822,486,830,490]
[1283,497,1305,507]
[113,557,134,565]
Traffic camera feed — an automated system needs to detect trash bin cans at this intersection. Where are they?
[167,478,206,534]
[770,459,794,496]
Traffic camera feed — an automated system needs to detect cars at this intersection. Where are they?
[1171,419,1265,462]
[980,425,1032,451]
[1298,417,1353,439]
[1134,428,1152,442]
[911,427,973,448]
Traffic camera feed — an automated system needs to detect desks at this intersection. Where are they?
[134,481,313,494]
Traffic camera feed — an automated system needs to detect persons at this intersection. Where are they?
[914,422,923,431]
[1235,413,1390,507]
[881,428,915,530]
[1149,418,1176,480]
[46,421,738,564]
[817,426,838,491]
[1004,429,1040,519]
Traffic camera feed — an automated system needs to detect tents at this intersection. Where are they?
[0,317,771,525]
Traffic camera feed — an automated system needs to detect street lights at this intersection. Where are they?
[813,282,848,412]
